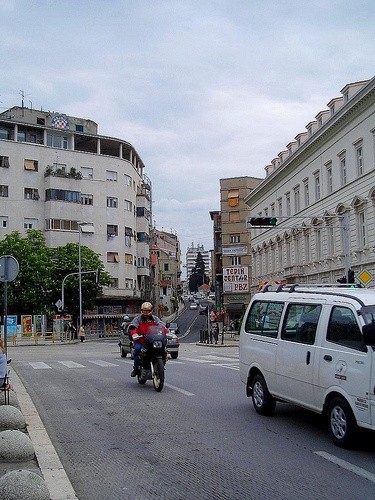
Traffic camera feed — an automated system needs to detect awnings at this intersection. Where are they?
[83,314,140,318]
[226,303,245,312]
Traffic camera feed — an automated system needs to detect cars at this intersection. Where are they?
[118,323,181,360]
[183,292,216,315]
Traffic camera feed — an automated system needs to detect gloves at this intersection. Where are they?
[166,333,173,339]
[132,333,139,341]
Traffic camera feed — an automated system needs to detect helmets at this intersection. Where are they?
[124,316,129,320]
[140,302,154,318]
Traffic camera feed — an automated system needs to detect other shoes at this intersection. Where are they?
[131,367,138,377]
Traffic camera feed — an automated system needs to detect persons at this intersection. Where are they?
[64,321,76,340]
[78,325,85,342]
[0,339,7,387]
[211,326,218,344]
[130,302,173,377]
[120,316,130,332]
[230,319,240,341]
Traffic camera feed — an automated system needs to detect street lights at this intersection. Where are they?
[76,220,88,341]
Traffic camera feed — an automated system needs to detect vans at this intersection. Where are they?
[237,281,375,450]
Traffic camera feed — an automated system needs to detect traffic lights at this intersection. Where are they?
[349,285,355,288]
[336,277,346,283]
[348,270,354,283]
[250,217,277,226]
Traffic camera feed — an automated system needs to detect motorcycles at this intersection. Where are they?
[128,323,171,392]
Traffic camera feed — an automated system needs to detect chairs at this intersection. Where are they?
[297,315,356,346]
